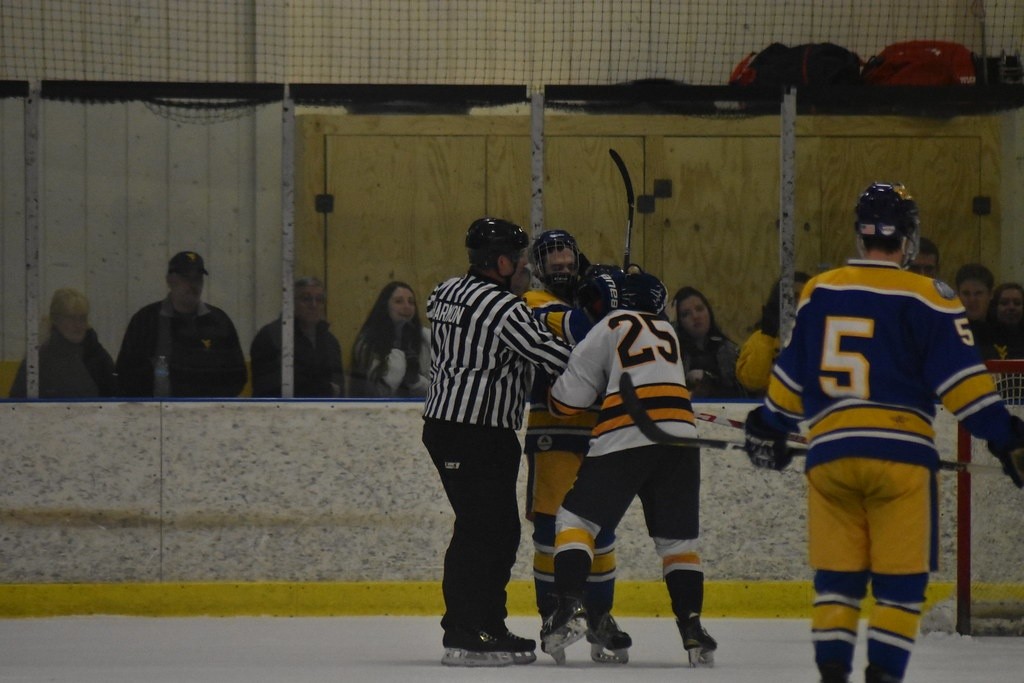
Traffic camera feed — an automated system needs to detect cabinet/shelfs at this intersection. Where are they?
[295,114,1000,398]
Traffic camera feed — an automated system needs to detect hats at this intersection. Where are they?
[168,248,208,276]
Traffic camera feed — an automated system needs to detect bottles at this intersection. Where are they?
[153,356,171,397]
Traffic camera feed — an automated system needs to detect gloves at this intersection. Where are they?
[988,414,1024,489]
[577,273,669,324]
[737,402,807,474]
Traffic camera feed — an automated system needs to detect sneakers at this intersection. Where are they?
[584,612,634,668]
[538,597,589,655]
[438,624,540,666]
[674,613,721,667]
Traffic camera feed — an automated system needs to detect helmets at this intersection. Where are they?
[584,262,626,283]
[459,214,531,260]
[530,228,581,292]
[857,179,921,270]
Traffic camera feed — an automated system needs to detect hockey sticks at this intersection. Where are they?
[606,144,638,273]
[692,411,808,446]
[617,369,1010,476]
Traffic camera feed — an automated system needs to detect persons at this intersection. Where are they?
[348,281,431,398]
[905,238,940,277]
[421,217,575,667]
[8,288,125,399]
[743,182,1024,683]
[250,276,346,398]
[665,287,753,399]
[955,263,1009,363]
[520,230,632,665]
[540,263,717,668]
[115,251,248,398]
[993,283,1024,359]
[734,271,812,399]
